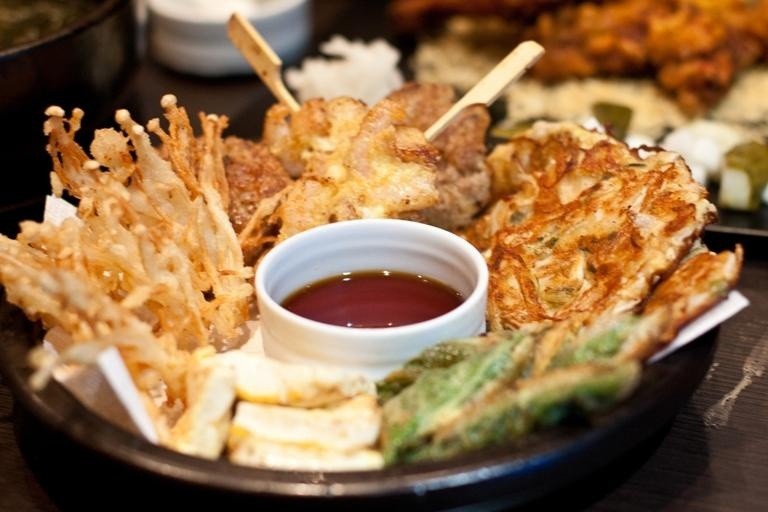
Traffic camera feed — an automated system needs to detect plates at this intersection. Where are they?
[2,285,729,510]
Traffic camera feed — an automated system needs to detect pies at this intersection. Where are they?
[482,119,745,361]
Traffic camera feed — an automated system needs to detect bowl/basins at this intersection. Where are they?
[254,215,490,367]
[135,0,309,78]
[0,0,131,130]
